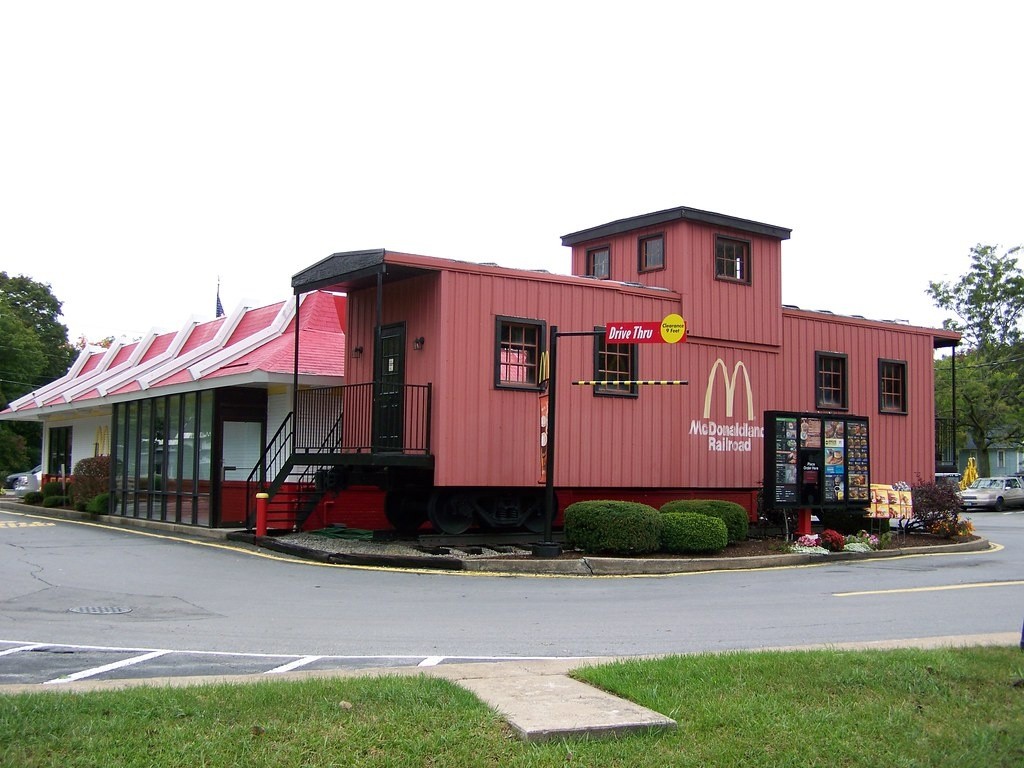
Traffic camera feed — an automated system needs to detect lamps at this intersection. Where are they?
[413,337,425,350]
[351,345,363,358]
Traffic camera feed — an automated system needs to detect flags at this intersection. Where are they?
[216,291,225,318]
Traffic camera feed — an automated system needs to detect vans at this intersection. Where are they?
[935,472,963,491]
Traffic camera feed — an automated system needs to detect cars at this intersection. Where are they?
[955,475,1024,512]
[15,471,42,498]
[5,464,43,490]
